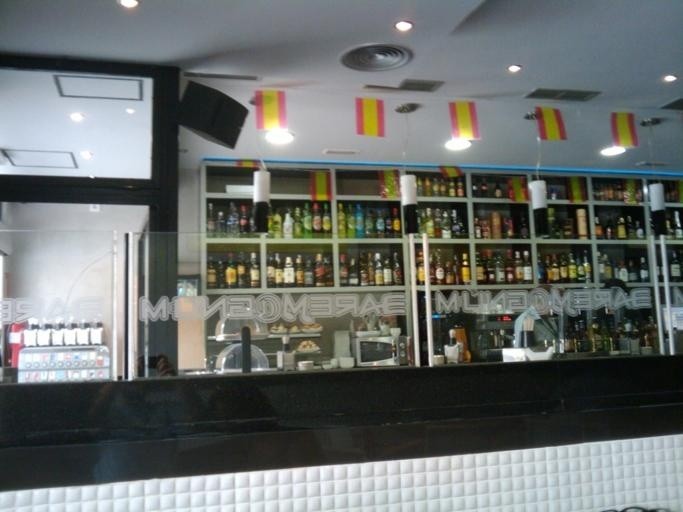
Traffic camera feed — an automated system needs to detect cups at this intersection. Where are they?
[434,355,444,364]
[448,329,456,345]
[488,334,504,348]
[520,329,534,347]
[380,324,389,336]
[390,327,401,336]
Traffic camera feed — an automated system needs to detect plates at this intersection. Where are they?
[356,331,380,336]
[295,357,354,371]
[270,328,324,335]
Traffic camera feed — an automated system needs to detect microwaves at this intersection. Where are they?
[350,337,407,366]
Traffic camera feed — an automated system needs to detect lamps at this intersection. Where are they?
[524,111,551,240]
[638,117,668,237]
[395,103,422,236]
[249,93,272,235]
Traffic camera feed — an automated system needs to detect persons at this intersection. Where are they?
[594,278,654,347]
[513,284,573,354]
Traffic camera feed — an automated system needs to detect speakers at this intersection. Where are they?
[178,81,249,149]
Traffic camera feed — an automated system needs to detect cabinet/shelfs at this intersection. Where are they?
[403,162,654,290]
[641,169,682,286]
[191,154,405,294]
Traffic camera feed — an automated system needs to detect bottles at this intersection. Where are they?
[281,334,289,352]
[204,180,683,288]
[562,309,669,356]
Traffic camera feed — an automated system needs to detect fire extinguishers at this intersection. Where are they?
[5,322,27,369]
